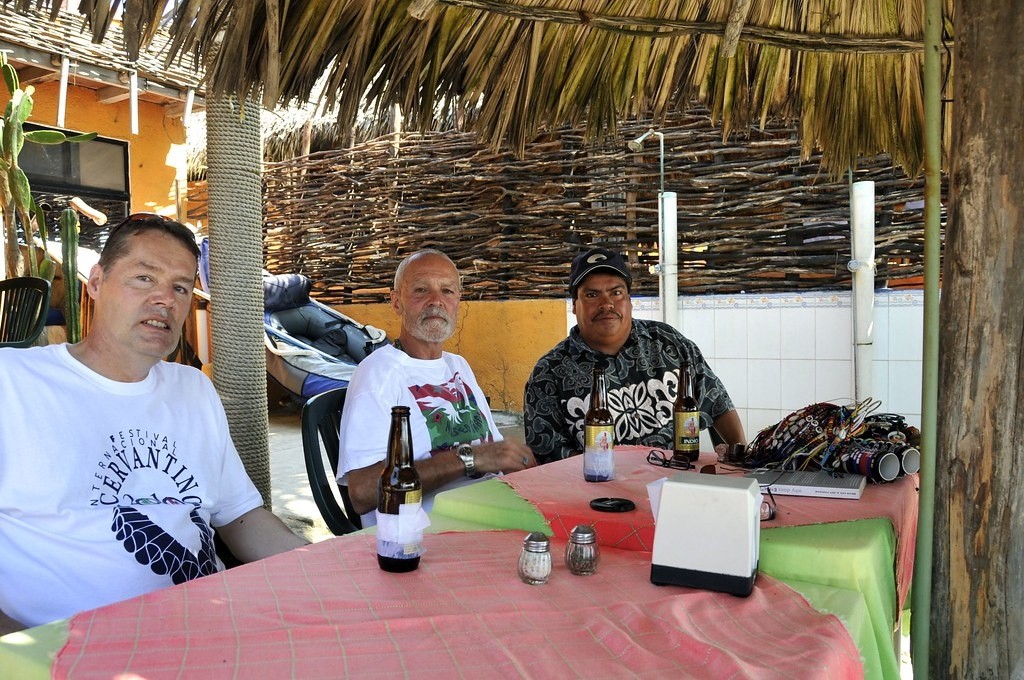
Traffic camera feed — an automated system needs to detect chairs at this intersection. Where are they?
[0,277,51,348]
[302,388,363,536]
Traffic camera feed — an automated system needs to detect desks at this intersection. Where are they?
[0,513,886,680]
[431,445,920,680]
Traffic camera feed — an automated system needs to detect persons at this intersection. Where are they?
[0,211,316,628]
[523,248,750,465]
[333,250,539,529]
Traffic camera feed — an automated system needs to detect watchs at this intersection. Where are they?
[456,443,476,477]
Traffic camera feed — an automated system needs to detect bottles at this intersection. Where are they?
[566,525,597,577]
[674,362,700,462]
[375,406,423,572]
[583,368,614,482]
[518,532,552,586]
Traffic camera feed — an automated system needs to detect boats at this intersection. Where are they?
[198,235,392,404]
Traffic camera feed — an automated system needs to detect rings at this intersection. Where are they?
[523,457,528,464]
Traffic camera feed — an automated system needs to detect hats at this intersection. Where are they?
[569,248,633,288]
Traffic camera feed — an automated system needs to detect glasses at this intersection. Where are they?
[760,486,777,521]
[646,449,695,471]
[101,213,174,254]
